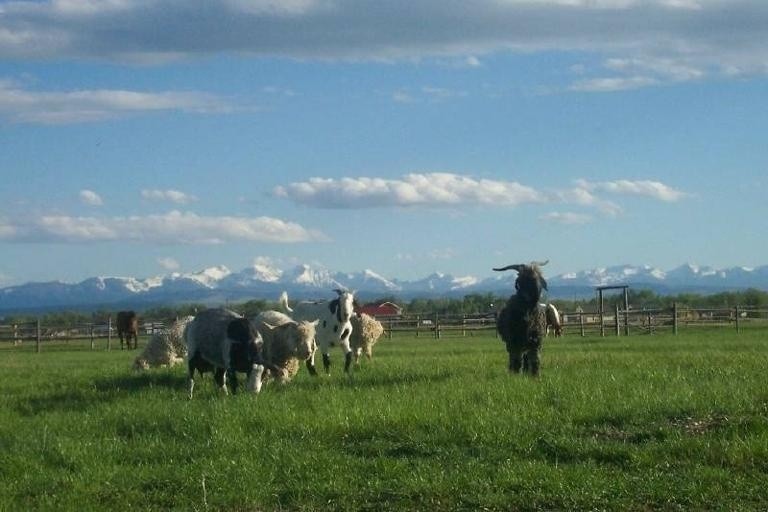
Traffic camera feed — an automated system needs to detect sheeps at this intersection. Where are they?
[491,257,550,380]
[184,287,385,399]
[130,315,195,372]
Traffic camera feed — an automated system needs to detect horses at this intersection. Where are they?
[544,302,564,340]
[116,310,139,350]
